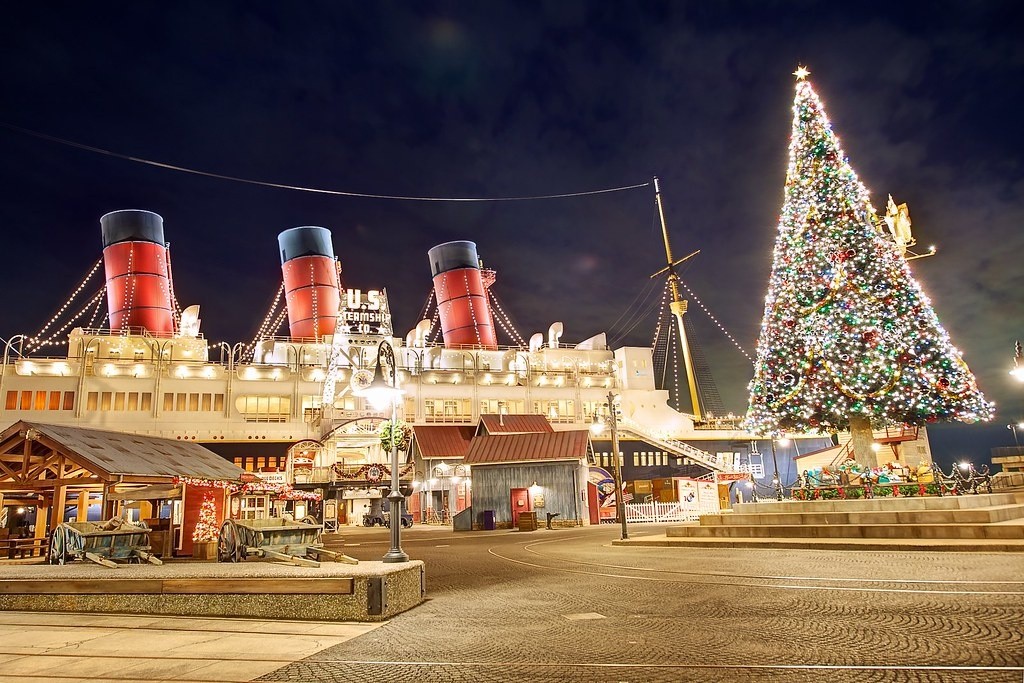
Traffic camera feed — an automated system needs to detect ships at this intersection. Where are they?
[0,143,845,527]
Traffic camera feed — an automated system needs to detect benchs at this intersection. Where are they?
[11,545,49,559]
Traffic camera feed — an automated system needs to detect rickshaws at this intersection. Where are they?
[46,516,163,568]
[216,514,361,568]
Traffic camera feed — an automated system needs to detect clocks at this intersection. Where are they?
[350,369,375,395]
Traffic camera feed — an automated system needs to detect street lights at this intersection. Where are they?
[590,392,630,539]
[364,339,408,562]
[1007,422,1024,446]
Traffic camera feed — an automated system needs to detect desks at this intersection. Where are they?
[1,537,50,559]
